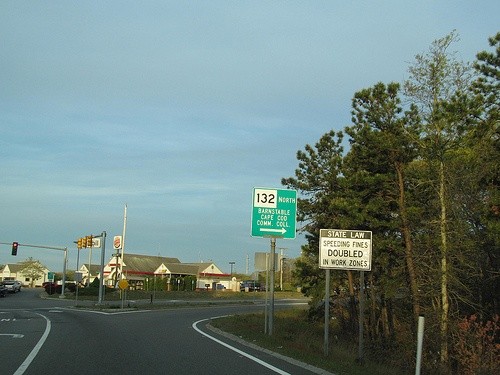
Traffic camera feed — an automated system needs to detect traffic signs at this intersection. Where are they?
[251,186,297,239]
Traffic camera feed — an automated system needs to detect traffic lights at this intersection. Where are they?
[87,234,92,247]
[82,236,87,248]
[12,242,18,256]
[77,237,81,248]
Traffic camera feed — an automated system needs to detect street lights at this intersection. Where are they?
[228,261,236,280]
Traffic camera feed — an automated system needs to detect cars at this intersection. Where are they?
[2,280,21,293]
[0,287,8,297]
